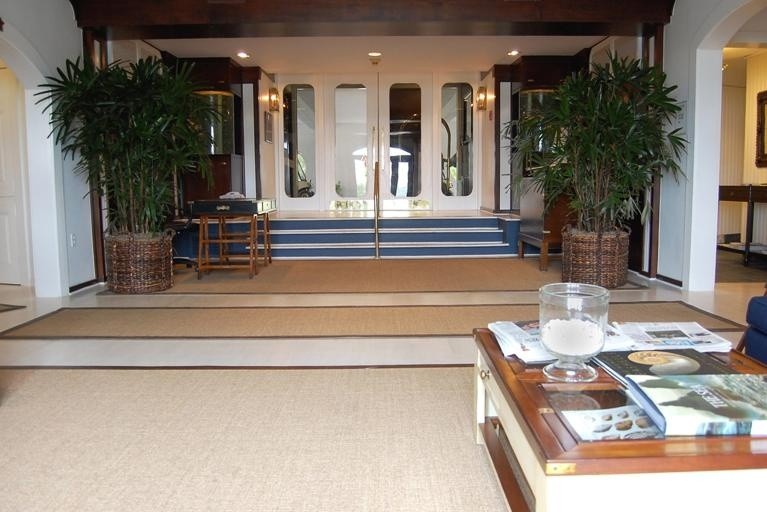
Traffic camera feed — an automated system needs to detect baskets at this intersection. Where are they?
[102,228,176,294]
[560,223,629,290]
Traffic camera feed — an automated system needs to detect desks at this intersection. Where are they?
[190,208,276,279]
[718,184,767,267]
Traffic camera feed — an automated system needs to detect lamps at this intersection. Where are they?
[476,87,486,110]
[269,87,281,111]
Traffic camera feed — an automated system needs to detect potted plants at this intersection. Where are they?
[496,48,690,290]
[33,55,230,295]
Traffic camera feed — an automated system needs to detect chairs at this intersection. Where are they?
[735,281,767,366]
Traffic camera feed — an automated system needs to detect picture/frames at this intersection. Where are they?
[755,90,767,167]
[265,110,273,143]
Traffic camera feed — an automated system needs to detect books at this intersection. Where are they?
[487,321,767,443]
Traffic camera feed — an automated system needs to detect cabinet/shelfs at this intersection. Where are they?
[169,57,243,215]
[511,56,589,272]
